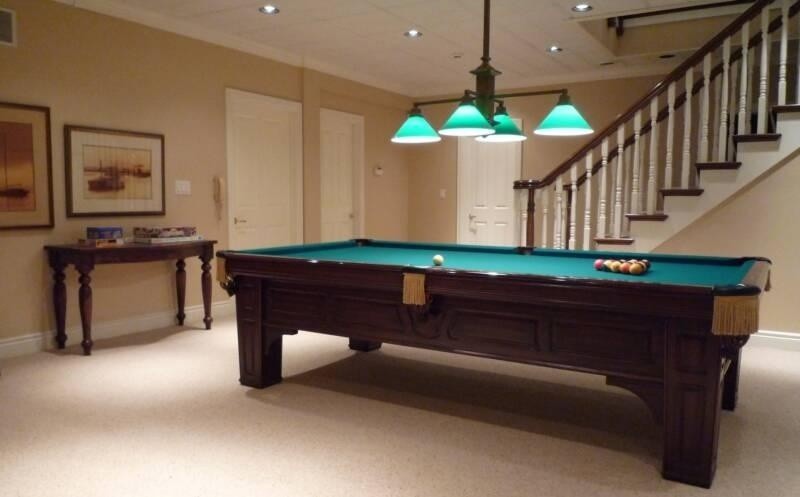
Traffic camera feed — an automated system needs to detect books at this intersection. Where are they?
[133,236,202,245]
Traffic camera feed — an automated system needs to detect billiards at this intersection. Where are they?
[594,258,649,274]
[433,255,444,265]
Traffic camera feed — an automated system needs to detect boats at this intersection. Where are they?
[128,166,151,178]
[88,167,125,191]
[1,188,30,198]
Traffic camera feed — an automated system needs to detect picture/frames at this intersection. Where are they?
[0,103,165,230]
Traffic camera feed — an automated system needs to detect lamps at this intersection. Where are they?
[390,1,595,144]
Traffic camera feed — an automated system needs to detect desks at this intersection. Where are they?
[43,239,218,356]
[215,239,773,490]
